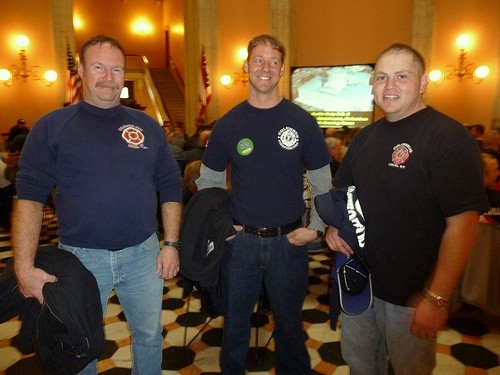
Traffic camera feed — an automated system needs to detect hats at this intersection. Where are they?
[331,251,375,317]
[18,119,25,125]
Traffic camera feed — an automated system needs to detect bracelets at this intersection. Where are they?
[163,239,183,251]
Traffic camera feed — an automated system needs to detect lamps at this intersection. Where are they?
[220,48,251,90]
[428,31,489,87]
[0,34,58,87]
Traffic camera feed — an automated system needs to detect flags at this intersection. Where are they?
[198,46,212,118]
[64,43,83,105]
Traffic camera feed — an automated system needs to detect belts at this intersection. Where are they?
[232,216,303,239]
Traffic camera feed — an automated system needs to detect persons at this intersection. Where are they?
[194,34,333,375]
[324,43,492,375]
[10,34,183,375]
[0,101,71,231]
[162,116,364,329]
[466,122,500,222]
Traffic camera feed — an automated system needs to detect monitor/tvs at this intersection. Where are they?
[289,64,375,130]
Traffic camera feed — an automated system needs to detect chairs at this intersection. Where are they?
[183,277,276,365]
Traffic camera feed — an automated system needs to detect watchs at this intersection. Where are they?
[421,288,451,311]
[313,229,323,238]
